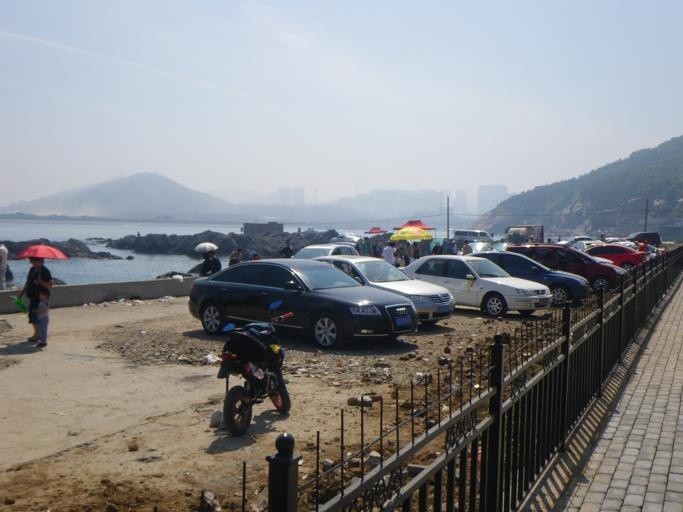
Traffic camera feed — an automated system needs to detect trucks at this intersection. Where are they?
[504,224,544,247]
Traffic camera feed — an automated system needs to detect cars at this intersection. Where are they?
[556,235,595,247]
[462,250,591,306]
[396,255,553,320]
[583,245,650,270]
[572,240,607,252]
[612,242,665,254]
[187,257,419,351]
[314,255,456,332]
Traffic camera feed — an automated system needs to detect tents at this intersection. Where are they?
[364,227,387,233]
[393,220,434,229]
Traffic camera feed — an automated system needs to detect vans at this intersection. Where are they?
[454,228,495,245]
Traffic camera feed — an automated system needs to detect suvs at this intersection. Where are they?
[618,231,662,247]
[287,242,359,262]
[507,242,627,297]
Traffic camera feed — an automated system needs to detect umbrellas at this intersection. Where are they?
[16,245,68,277]
[389,226,434,242]
[193,242,219,253]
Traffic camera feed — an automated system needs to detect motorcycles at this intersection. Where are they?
[216,312,295,438]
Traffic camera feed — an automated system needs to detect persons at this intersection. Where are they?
[34,288,52,348]
[381,243,398,265]
[251,252,259,260]
[279,240,294,258]
[404,243,411,266]
[412,242,419,260]
[19,257,53,342]
[631,241,639,250]
[229,247,243,266]
[640,240,649,251]
[431,242,443,255]
[463,240,473,256]
[0,240,9,290]
